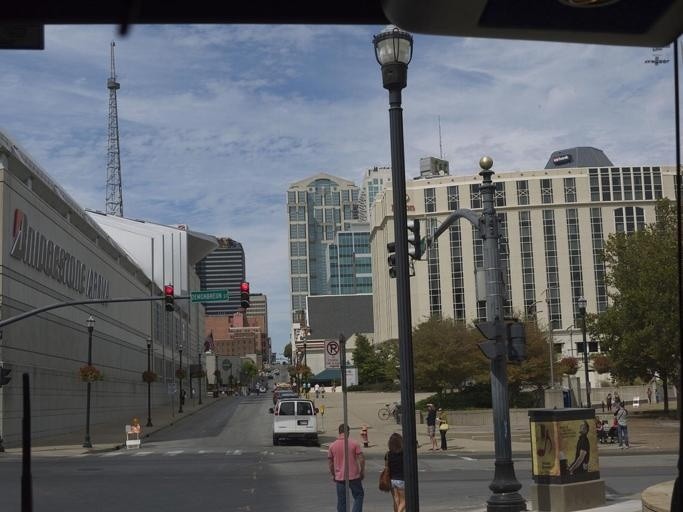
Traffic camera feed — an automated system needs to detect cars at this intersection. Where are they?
[256,360,319,446]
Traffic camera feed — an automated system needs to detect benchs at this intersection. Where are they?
[597,431,613,444]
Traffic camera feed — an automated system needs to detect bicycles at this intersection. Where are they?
[377,401,399,420]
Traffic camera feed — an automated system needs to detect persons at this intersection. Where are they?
[255,379,339,398]
[436,406,448,451]
[569,421,590,475]
[425,403,437,450]
[327,423,364,512]
[647,388,652,404]
[595,391,630,449]
[384,433,406,512]
[182,388,186,405]
[655,388,659,403]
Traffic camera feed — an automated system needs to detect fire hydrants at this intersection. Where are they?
[360,426,370,448]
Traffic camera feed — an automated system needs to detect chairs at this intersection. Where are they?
[126,425,140,450]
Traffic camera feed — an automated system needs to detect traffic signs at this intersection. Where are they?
[189,289,229,302]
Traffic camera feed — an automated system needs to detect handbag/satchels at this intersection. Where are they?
[379,451,391,491]
[439,421,448,431]
[613,417,618,425]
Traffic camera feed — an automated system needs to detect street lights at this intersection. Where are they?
[177,343,184,413]
[295,349,300,395]
[576,294,592,406]
[144,335,152,427]
[83,314,95,448]
[301,319,311,399]
[197,344,203,404]
[373,23,429,511]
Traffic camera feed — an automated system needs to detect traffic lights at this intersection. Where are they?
[407,218,423,260]
[474,319,502,362]
[164,285,174,311]
[0,366,11,390]
[385,240,395,279]
[240,281,249,309]
